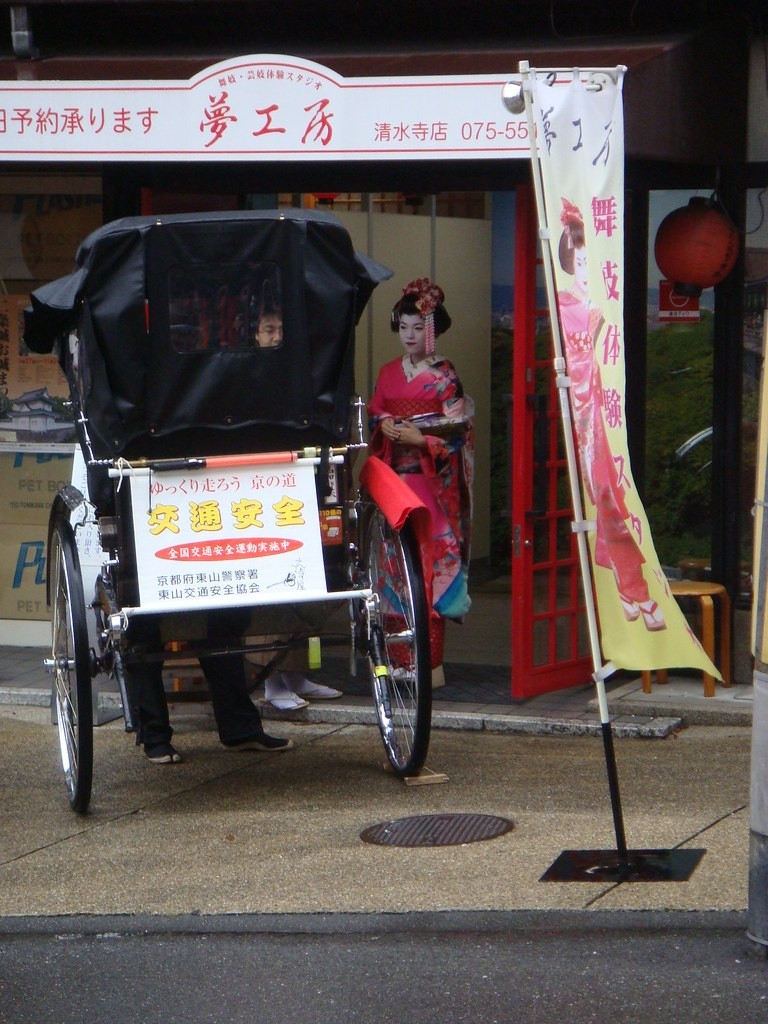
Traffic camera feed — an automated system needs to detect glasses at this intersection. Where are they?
[258,328,282,336]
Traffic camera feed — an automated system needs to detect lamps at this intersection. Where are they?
[502,71,557,114]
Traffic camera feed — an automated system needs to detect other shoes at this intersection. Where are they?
[392,666,445,691]
[143,743,181,763]
[222,731,293,752]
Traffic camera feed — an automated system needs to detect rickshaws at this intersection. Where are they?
[14,206,449,819]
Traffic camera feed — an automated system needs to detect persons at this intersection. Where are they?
[243,297,343,710]
[367,282,475,690]
[129,653,294,763]
[554,221,668,632]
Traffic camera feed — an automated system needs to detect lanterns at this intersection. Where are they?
[654,196,739,297]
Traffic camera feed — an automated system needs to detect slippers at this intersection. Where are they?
[290,680,342,699]
[640,600,666,631]
[259,692,309,710]
[620,590,640,621]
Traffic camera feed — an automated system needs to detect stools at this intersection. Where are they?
[640,580,730,698]
[680,559,749,581]
